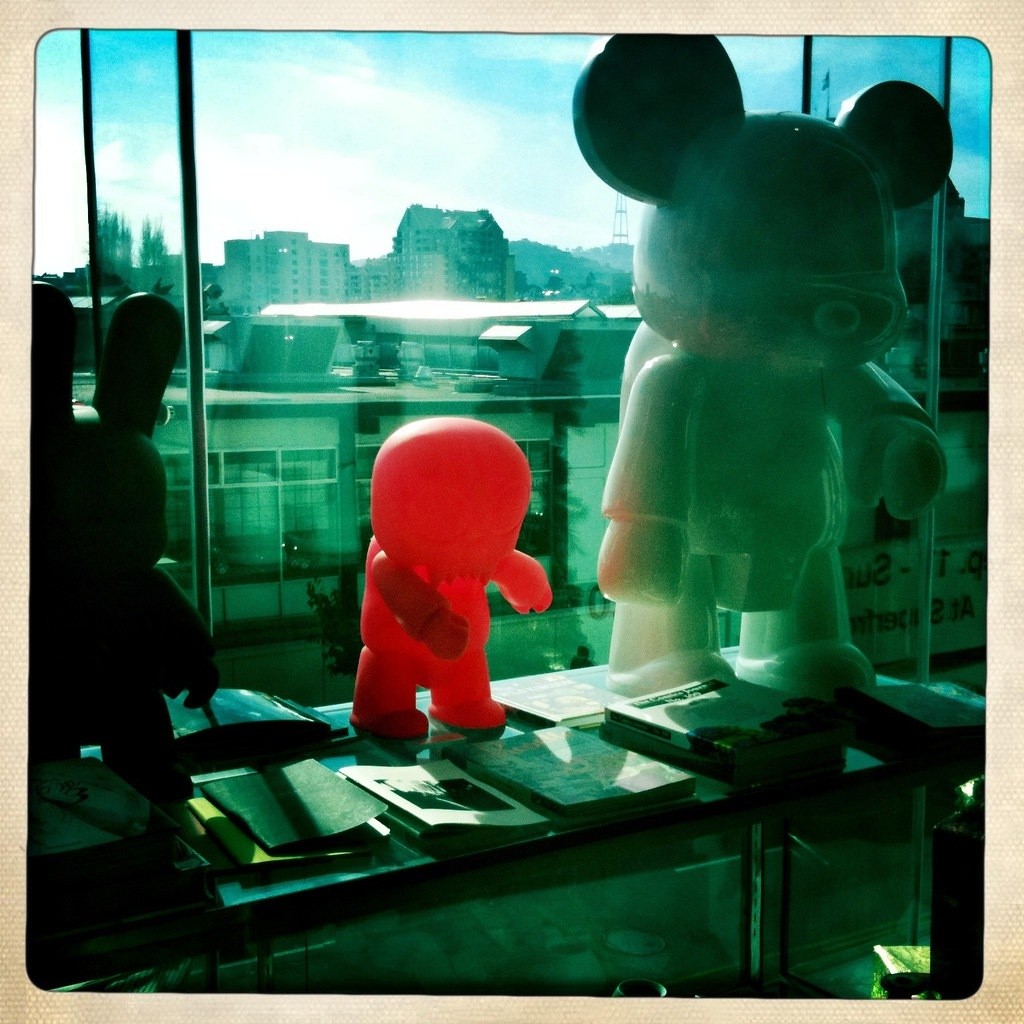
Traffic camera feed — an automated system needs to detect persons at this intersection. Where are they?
[567,643,597,668]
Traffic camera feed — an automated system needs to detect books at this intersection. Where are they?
[26,686,553,936]
[487,673,607,727]
[522,795,701,827]
[597,722,846,785]
[440,724,697,817]
[603,669,859,766]
[827,675,984,765]
